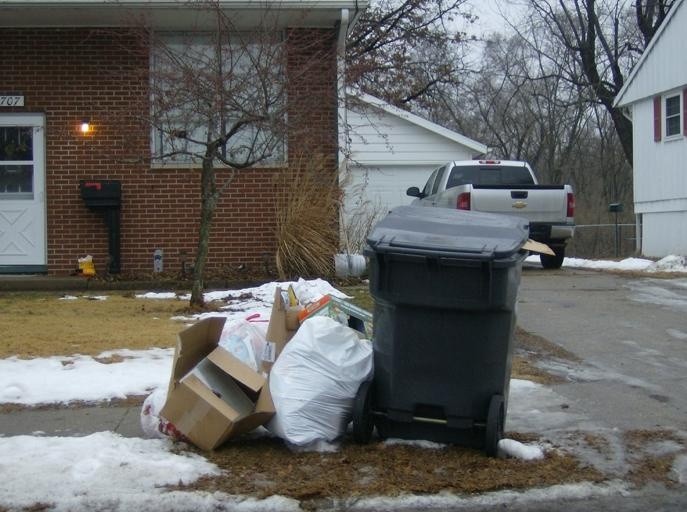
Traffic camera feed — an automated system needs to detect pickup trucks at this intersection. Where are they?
[405,159,576,268]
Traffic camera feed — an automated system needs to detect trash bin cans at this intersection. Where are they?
[351,204,530,458]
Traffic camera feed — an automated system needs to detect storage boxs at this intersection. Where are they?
[262,287,300,372]
[161,316,278,452]
[299,293,375,341]
